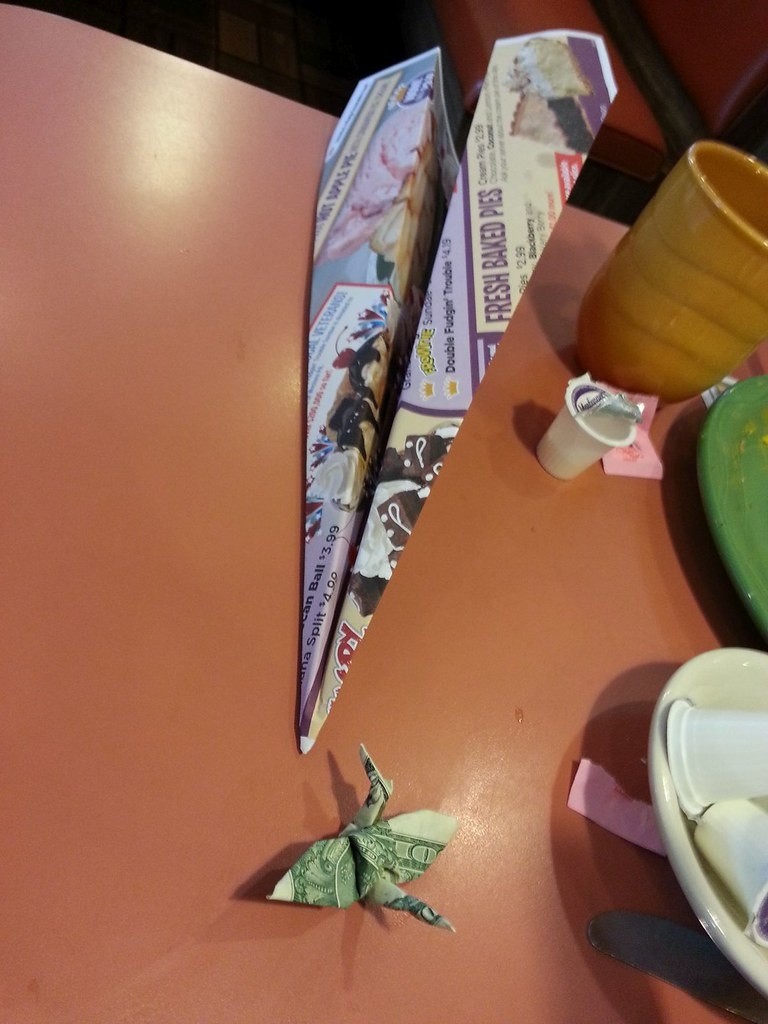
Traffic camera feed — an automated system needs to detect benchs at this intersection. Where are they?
[407,0,768,220]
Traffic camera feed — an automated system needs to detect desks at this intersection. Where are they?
[0,0,768,1024]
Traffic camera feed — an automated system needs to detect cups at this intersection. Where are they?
[694,799,768,949]
[537,382,637,480]
[579,140,768,407]
[667,700,768,815]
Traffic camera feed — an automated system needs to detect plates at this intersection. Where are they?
[648,648,768,996]
[699,376,768,640]
